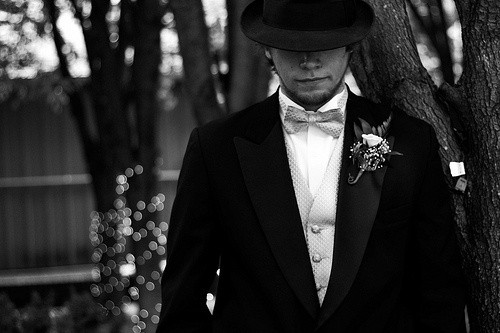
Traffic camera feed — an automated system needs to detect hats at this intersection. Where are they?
[240,0,376,53]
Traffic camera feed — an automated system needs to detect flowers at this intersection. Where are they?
[346,111,404,184]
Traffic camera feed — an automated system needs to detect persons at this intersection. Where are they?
[154,2,484,331]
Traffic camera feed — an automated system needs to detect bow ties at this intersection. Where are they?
[283,106,344,138]
